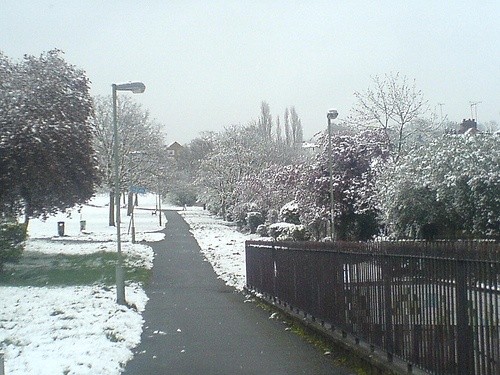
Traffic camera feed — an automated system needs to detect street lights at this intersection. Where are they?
[327,110,338,239]
[112,82,146,252]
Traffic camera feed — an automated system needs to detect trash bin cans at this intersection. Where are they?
[57,222,64,236]
[80,220,86,231]
